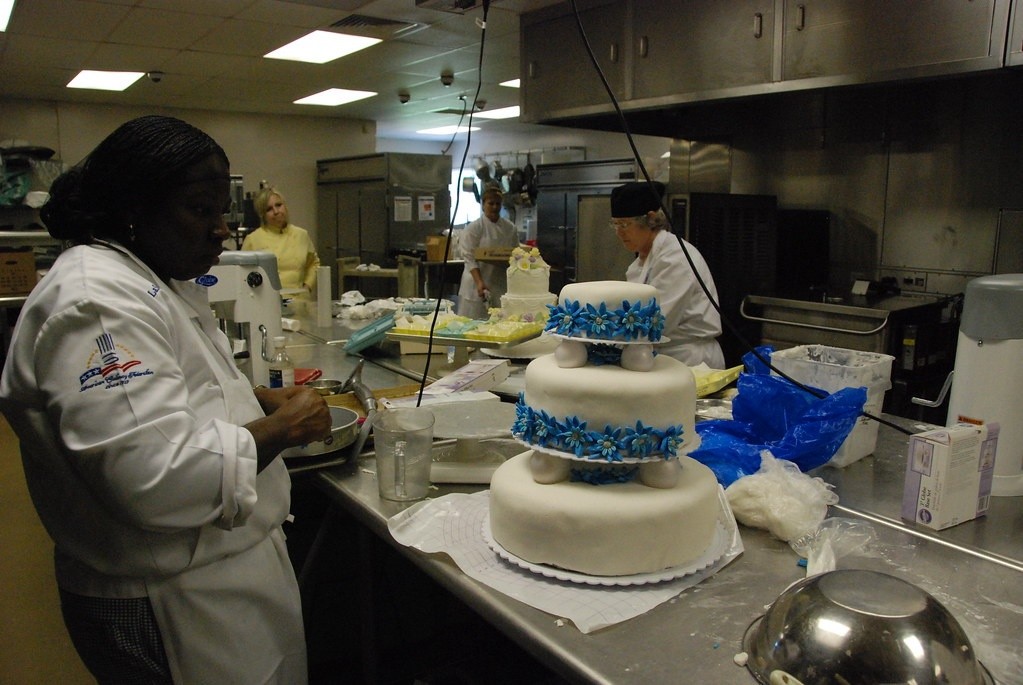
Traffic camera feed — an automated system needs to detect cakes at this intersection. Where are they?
[487,282,718,578]
[499,243,558,321]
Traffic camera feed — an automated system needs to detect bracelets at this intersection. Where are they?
[253,384,269,391]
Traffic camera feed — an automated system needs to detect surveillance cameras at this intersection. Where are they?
[441,76,454,87]
[148,71,164,83]
[476,101,486,109]
[398,95,410,103]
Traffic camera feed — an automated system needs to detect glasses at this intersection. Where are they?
[606,218,649,230]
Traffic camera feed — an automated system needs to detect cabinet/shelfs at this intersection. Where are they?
[516,0,1020,126]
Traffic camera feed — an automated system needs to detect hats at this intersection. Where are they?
[482,190,502,203]
[610,180,667,217]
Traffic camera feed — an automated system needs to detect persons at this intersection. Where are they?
[0,113,333,685]
[241,188,320,301]
[458,189,520,320]
[610,181,725,370]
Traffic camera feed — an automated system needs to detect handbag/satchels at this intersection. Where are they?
[685,344,867,490]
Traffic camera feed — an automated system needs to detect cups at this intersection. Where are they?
[371,407,435,501]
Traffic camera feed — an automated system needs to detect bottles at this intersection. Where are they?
[268,336,295,388]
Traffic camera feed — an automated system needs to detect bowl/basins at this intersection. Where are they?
[279,405,359,458]
[304,380,342,396]
[742,569,998,685]
